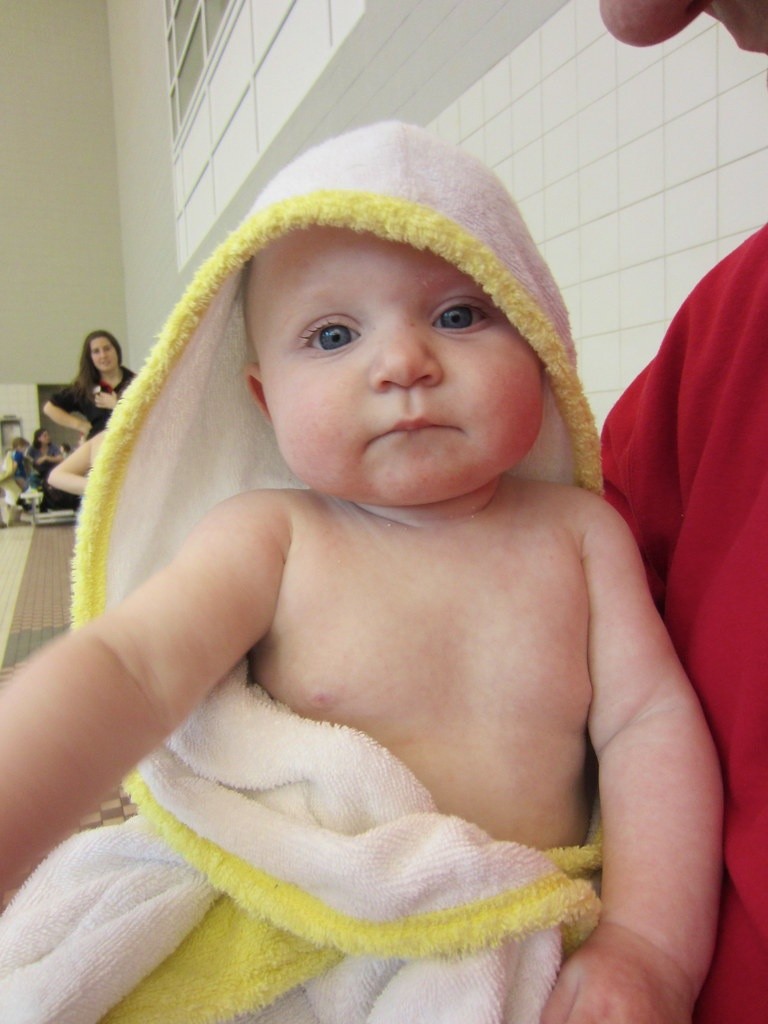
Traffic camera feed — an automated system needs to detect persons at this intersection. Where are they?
[12,437,29,488]
[598,0,768,1024]
[0,122,726,1024]
[60,443,71,459]
[46,430,104,496]
[42,330,137,440]
[25,428,62,479]
